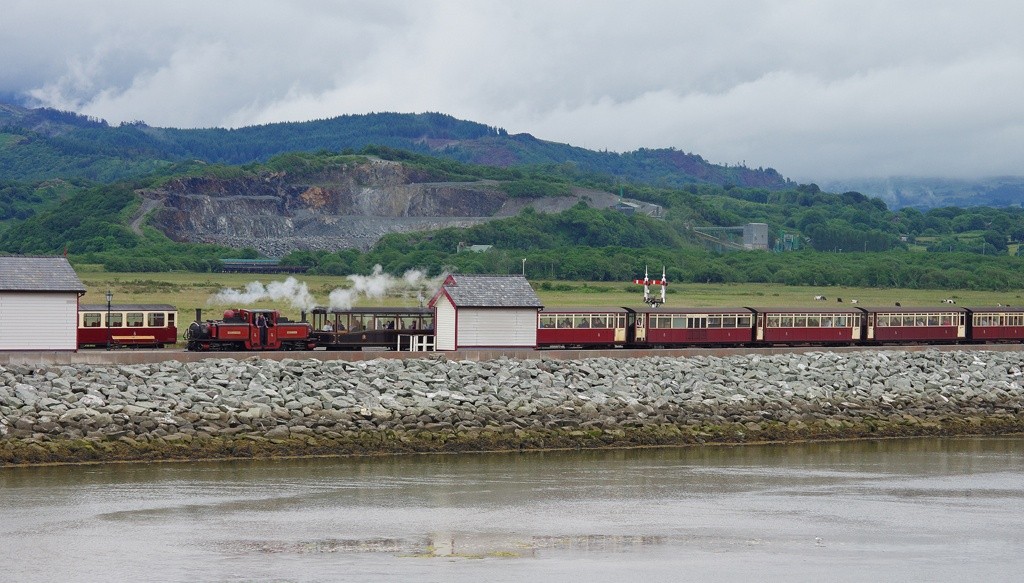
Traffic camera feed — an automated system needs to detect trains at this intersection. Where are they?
[182,306,1024,352]
[77,304,178,349]
[217,258,311,274]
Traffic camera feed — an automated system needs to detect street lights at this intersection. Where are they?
[418,295,426,307]
[105,290,114,350]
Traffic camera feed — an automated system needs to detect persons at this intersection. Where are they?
[978,316,1022,325]
[322,319,434,331]
[758,316,841,327]
[540,318,604,328]
[255,313,269,346]
[878,316,951,326]
[637,318,642,325]
